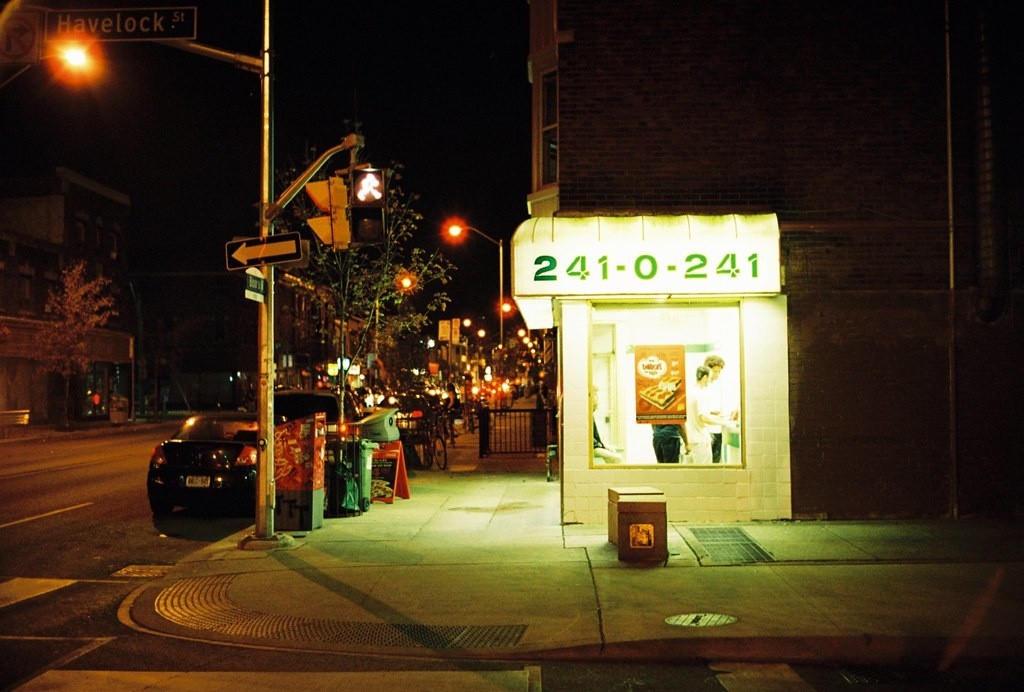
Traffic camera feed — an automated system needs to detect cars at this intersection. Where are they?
[272,389,364,462]
[146,412,261,524]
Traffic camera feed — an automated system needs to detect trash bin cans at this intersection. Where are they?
[109,394,129,427]
[331,439,380,512]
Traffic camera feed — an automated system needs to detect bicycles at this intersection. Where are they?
[394,397,495,471]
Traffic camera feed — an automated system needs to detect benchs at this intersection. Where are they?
[0,409,31,431]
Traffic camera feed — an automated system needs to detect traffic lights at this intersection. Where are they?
[304,177,350,255]
[355,167,384,205]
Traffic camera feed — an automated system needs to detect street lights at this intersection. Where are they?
[47,31,281,546]
[448,223,505,348]
[374,273,414,356]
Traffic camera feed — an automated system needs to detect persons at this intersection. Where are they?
[444,382,459,446]
[652,356,739,464]
[591,386,622,465]
